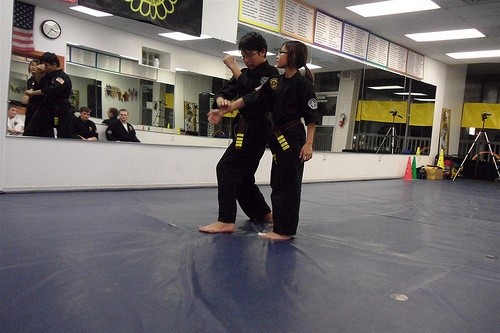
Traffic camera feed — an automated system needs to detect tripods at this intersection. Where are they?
[453,121,500,182]
[375,116,401,155]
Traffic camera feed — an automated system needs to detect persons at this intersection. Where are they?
[199,30,318,239]
[7,51,141,142]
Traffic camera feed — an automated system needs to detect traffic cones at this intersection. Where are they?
[404,156,413,181]
[411,156,417,180]
[167,122,170,129]
[177,127,181,135]
[437,149,445,171]
[416,147,420,156]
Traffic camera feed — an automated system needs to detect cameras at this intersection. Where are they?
[482,113,491,121]
[390,110,397,116]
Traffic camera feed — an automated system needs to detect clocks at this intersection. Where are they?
[40,19,62,39]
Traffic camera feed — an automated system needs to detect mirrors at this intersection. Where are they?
[66,63,175,130]
[5,0,436,156]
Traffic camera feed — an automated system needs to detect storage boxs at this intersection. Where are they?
[423,164,443,179]
